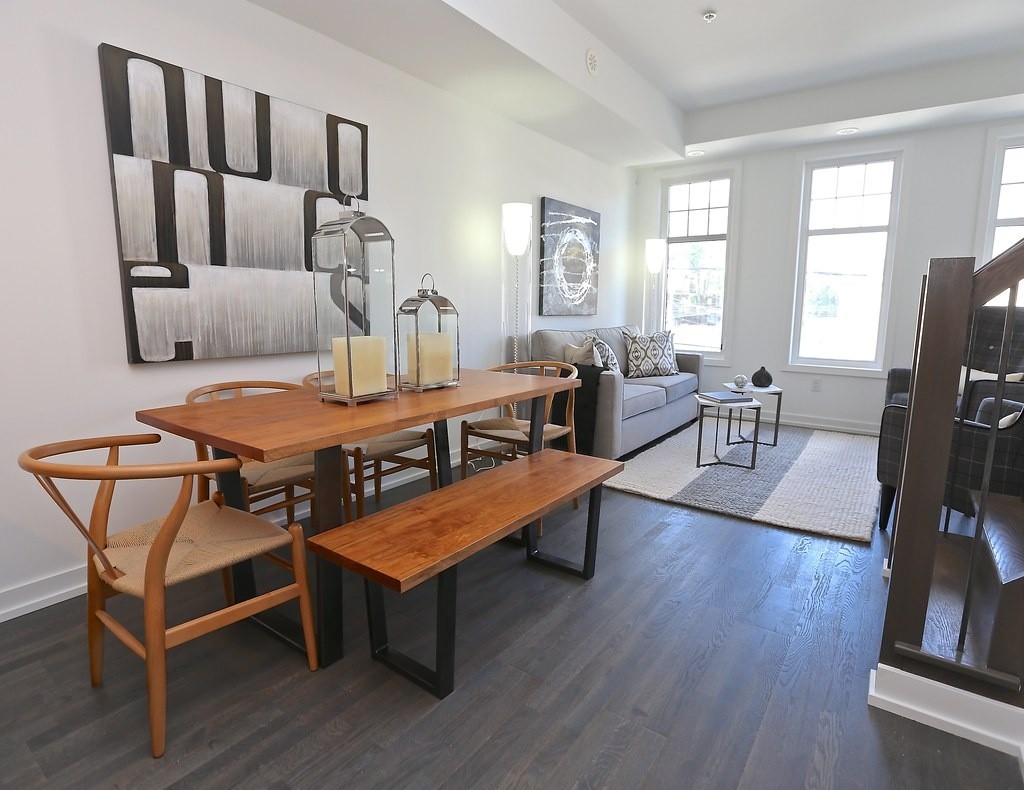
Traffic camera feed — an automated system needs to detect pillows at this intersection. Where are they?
[565,342,603,368]
[999,412,1020,428]
[622,330,679,378]
[670,332,680,372]
[958,366,1024,395]
[583,336,621,372]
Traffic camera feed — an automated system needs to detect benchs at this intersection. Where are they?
[308,448,625,700]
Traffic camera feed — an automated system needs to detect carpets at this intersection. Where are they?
[601,416,882,542]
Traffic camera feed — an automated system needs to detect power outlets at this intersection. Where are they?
[809,378,821,392]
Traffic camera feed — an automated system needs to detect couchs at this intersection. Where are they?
[885,306,1024,423]
[876,397,1024,529]
[508,324,705,459]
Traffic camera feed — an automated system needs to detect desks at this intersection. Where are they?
[135,367,583,668]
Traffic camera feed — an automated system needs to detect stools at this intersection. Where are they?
[694,395,762,470]
[723,383,783,447]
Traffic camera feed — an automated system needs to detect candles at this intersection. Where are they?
[406,331,452,386]
[332,336,387,397]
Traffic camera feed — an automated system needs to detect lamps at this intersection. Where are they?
[642,239,668,335]
[502,202,533,465]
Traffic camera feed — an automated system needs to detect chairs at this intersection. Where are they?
[186,381,352,603]
[460,360,578,537]
[303,370,436,520]
[17,434,319,759]
